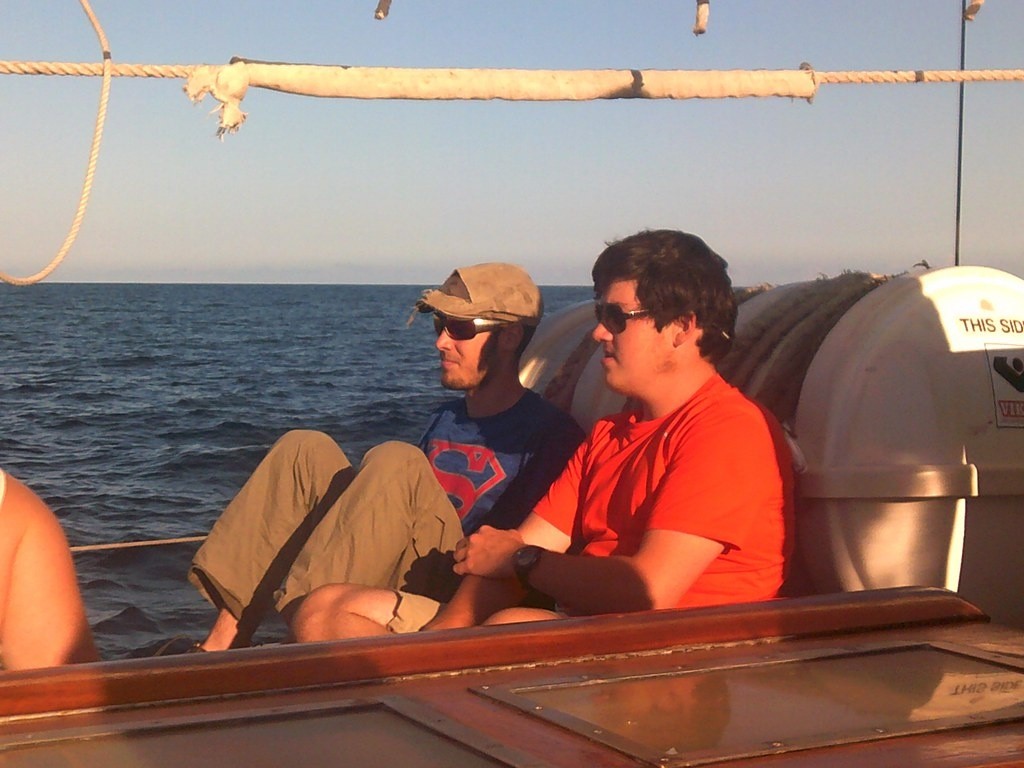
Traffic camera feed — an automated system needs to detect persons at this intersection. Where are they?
[0,469,102,672]
[185,260,588,652]
[292,230,797,641]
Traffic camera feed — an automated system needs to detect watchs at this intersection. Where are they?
[513,545,546,592]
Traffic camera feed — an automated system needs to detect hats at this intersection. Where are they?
[416,263,542,325]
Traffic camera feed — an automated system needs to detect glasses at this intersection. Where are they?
[595,304,649,334]
[434,313,510,339]
